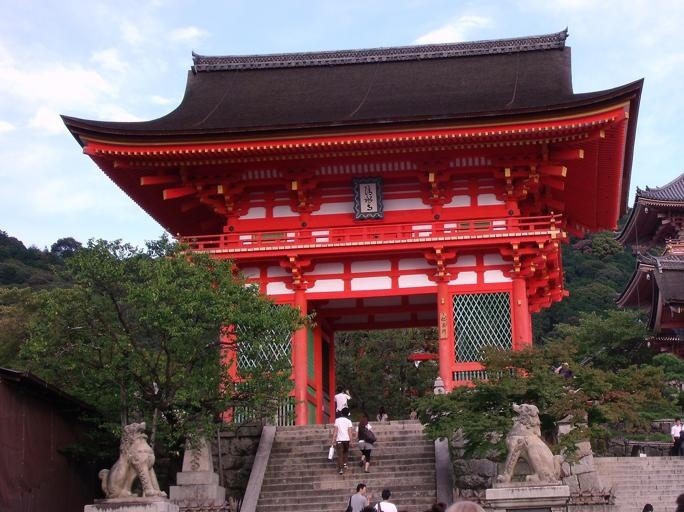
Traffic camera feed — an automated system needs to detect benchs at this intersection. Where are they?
[622,437,675,455]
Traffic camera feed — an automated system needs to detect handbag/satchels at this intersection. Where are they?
[326,443,336,461]
[345,495,355,512]
[363,430,377,444]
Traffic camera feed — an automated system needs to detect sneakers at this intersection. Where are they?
[338,462,348,476]
[359,461,370,474]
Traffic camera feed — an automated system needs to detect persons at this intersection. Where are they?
[437,502,446,512]
[670,417,683,455]
[375,407,389,424]
[358,505,378,511]
[554,362,574,379]
[346,482,373,512]
[641,503,654,512]
[329,406,355,474]
[372,488,399,512]
[444,500,486,512]
[332,386,352,417]
[431,503,443,512]
[355,416,376,473]
